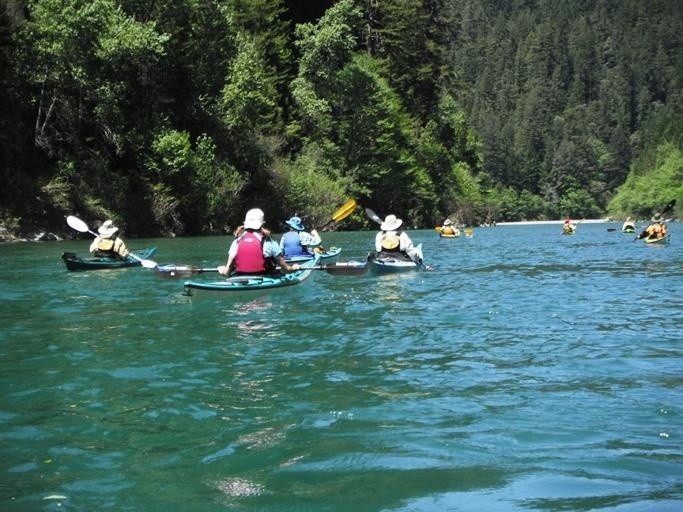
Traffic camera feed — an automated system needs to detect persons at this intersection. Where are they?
[86,219,128,261]
[277,216,322,260]
[372,214,420,261]
[642,212,667,240]
[439,218,459,235]
[216,207,300,278]
[622,216,635,230]
[561,218,576,232]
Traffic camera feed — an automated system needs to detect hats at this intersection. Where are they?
[284,215,304,231]
[243,207,265,231]
[649,212,664,223]
[442,219,451,225]
[97,219,119,235]
[379,214,403,232]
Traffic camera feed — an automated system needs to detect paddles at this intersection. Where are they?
[67,215,156,269]
[436,227,473,235]
[176,262,367,275]
[608,228,621,232]
[634,199,676,243]
[365,208,434,273]
[316,199,357,233]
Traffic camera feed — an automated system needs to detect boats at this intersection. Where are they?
[62,247,159,271]
[182,255,321,296]
[621,222,636,233]
[438,228,462,239]
[365,244,426,272]
[643,223,672,244]
[282,248,342,263]
[561,226,578,236]
